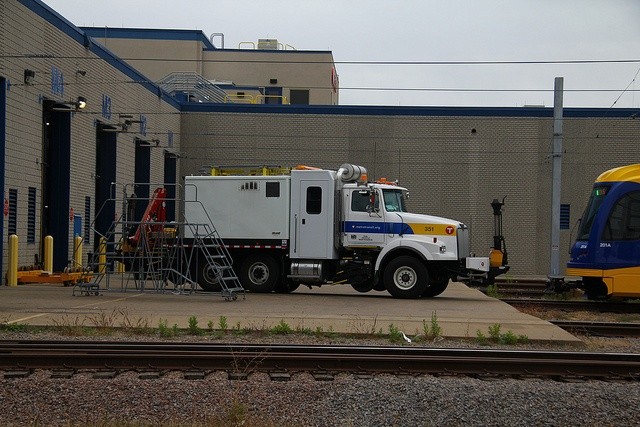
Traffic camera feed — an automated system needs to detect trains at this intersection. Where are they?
[566,163,640,300]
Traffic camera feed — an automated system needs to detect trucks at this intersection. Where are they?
[184,164,471,297]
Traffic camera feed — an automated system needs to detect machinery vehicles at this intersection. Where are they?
[124,187,177,279]
